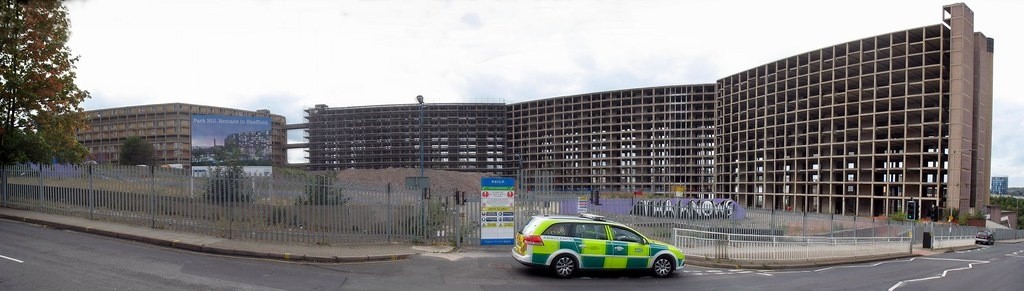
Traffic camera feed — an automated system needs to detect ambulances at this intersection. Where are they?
[511,213,687,278]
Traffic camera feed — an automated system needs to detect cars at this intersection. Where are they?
[975,230,995,245]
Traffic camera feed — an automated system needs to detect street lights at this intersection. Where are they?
[415,94,425,238]
